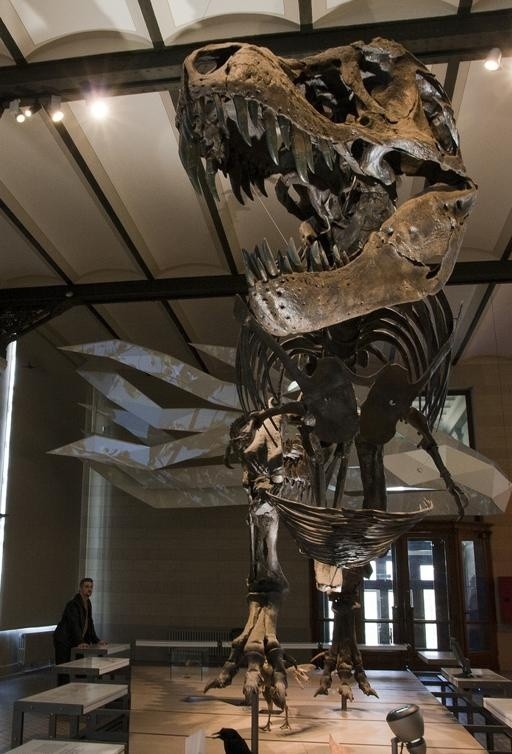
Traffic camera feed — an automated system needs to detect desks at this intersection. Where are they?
[134,633,410,668]
[414,645,511,753]
[1,643,132,752]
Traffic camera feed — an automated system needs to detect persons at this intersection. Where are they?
[53,578,106,686]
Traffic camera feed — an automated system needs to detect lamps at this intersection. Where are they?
[1,88,120,128]
[380,703,430,754]
[478,42,508,74]
